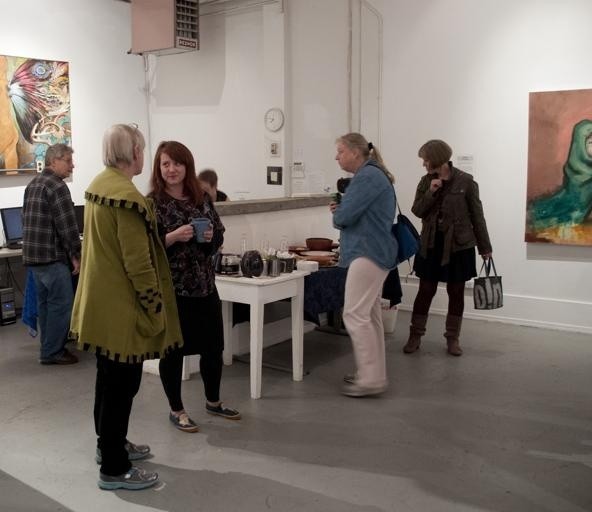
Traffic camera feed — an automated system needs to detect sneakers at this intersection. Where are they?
[97,466,159,490]
[169,408,201,432]
[95,440,150,462]
[340,373,387,397]
[206,400,241,420]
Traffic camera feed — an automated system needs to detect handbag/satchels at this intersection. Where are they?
[393,213,421,263]
[474,256,503,309]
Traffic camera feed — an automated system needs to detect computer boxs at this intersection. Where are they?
[0,286,17,327]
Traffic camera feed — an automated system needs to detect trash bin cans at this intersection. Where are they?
[382,306,398,333]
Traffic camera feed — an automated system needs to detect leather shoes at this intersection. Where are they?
[39,348,77,364]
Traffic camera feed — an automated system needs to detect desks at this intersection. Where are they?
[0,247,22,259]
[181,268,312,400]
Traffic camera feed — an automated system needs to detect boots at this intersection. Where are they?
[404,313,428,353]
[443,314,463,355]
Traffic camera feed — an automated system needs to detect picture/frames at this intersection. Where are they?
[266,167,281,185]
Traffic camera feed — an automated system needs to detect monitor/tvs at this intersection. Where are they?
[0,206,23,249]
[70,205,85,239]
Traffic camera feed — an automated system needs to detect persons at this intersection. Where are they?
[22,143,81,365]
[143,141,242,433]
[328,133,399,397]
[403,140,493,356]
[68,122,184,491]
[197,169,230,202]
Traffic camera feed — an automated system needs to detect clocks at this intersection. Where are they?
[264,107,284,132]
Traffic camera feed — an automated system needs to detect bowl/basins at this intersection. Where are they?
[306,238,333,250]
[300,251,336,261]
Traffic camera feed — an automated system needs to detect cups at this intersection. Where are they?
[190,218,211,242]
[262,258,296,276]
